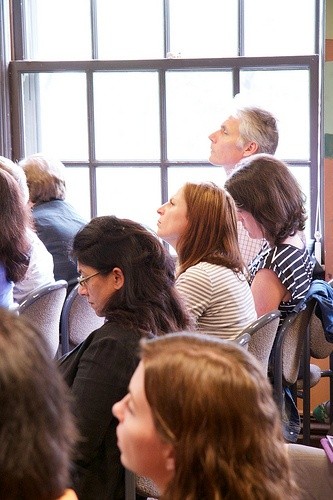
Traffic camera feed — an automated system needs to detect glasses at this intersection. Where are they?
[77,272,102,286]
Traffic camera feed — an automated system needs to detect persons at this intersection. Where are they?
[156,182,256,340]
[0,156,54,310]
[20,154,86,281]
[112,333,313,500]
[224,154,314,377]
[55,216,189,500]
[208,107,279,264]
[0,312,74,500]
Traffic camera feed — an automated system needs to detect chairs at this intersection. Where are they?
[16,278,331,442]
